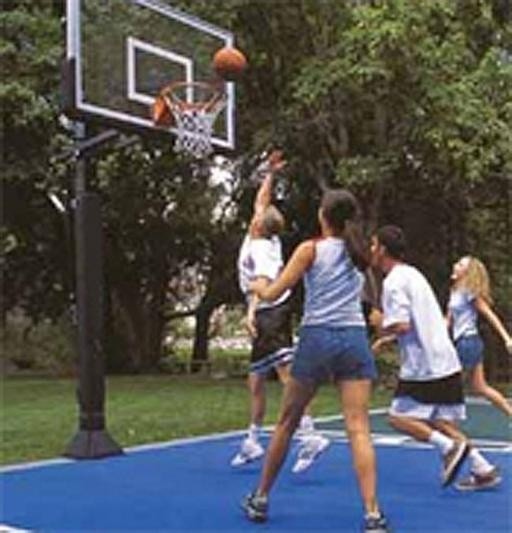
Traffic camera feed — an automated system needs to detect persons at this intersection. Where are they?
[368,224,502,492]
[236,188,393,532]
[444,254,512,415]
[231,152,331,474]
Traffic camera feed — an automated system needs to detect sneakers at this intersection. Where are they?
[230,437,265,467]
[239,491,268,521]
[453,465,501,489]
[439,437,471,486]
[290,428,332,474]
[362,510,391,532]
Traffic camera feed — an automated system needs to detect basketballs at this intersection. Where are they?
[213,46,247,75]
